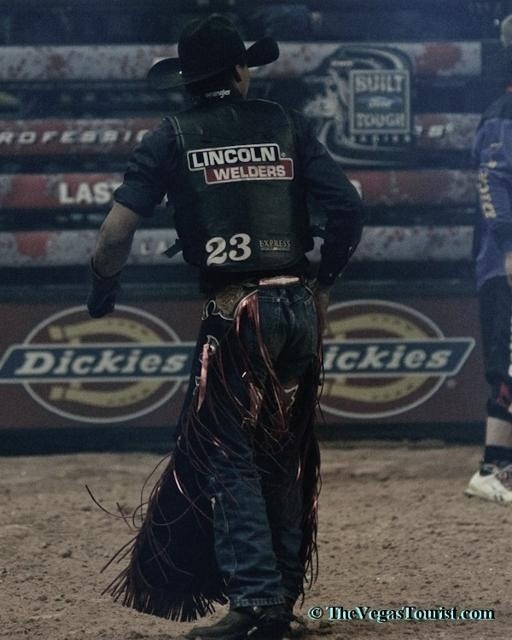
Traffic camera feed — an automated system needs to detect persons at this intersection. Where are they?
[83,15,366,640]
[461,14,512,507]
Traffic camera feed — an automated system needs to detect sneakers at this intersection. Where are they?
[464,470,511,505]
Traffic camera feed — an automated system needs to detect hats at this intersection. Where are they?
[146,14,279,91]
[500,15,511,46]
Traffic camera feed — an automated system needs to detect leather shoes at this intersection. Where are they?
[187,600,294,640]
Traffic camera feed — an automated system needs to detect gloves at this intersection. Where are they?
[315,285,329,329]
[87,257,127,318]
